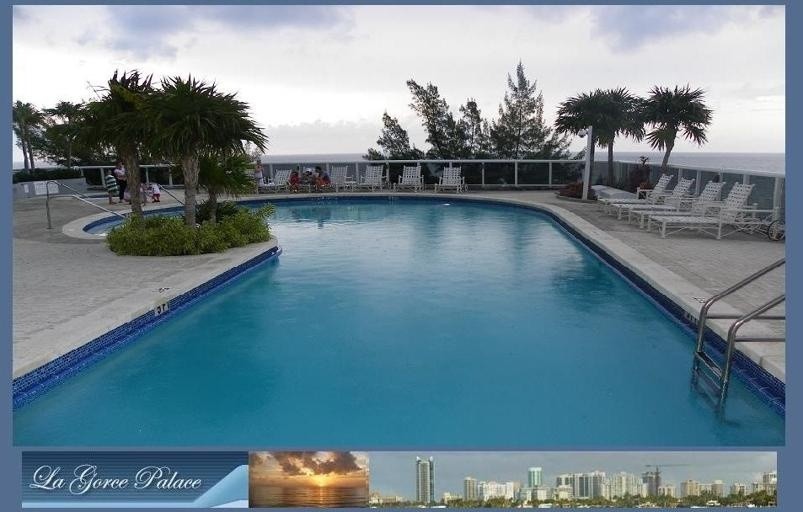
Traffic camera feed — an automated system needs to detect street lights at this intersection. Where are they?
[576,122,593,200]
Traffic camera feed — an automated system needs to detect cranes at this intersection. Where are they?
[644,461,689,497]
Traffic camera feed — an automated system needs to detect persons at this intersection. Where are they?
[286,165,331,193]
[254,160,264,194]
[639,177,652,199]
[104,158,161,206]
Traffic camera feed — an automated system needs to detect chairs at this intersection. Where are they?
[241,165,471,193]
[589,173,777,241]
